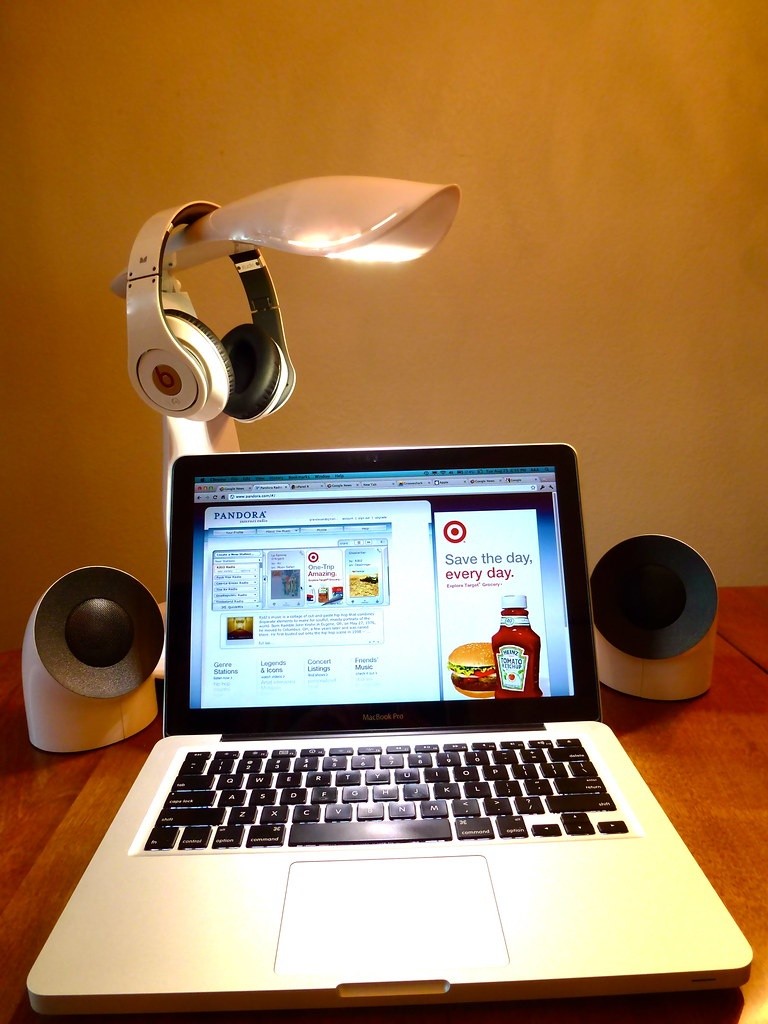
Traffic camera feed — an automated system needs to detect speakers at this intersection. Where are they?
[22,565,166,753]
[588,534,720,704]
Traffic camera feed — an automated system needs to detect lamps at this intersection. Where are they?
[109,174,459,676]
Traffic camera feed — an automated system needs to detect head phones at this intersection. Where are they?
[125,201,297,424]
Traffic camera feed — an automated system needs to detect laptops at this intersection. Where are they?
[26,442,754,1018]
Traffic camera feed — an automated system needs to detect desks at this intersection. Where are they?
[0,586,768,1024]
